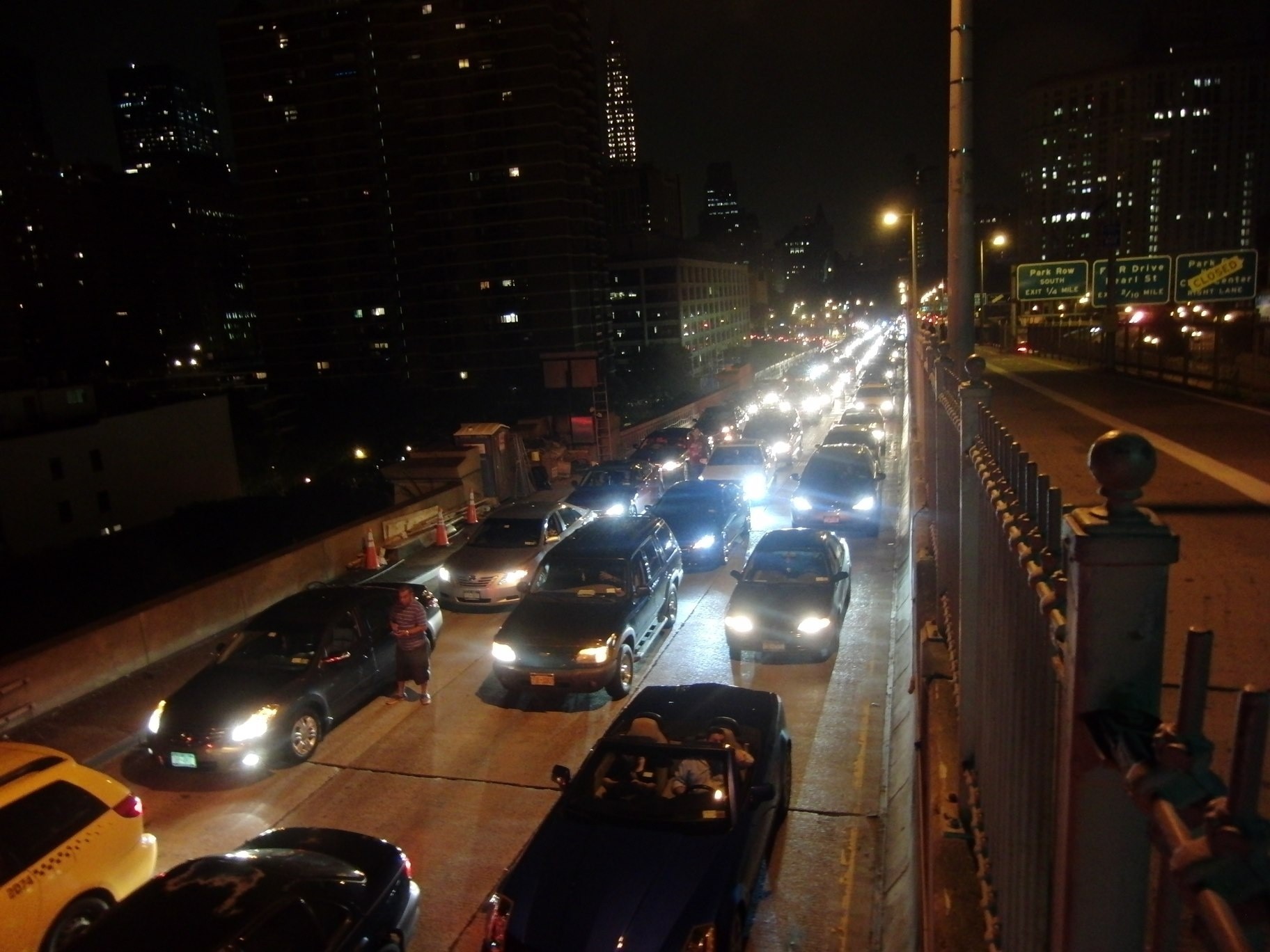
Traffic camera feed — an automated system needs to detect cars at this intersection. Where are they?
[0,740,160,952]
[144,579,444,776]
[697,439,780,505]
[479,682,796,952]
[439,502,599,607]
[725,526,853,666]
[787,441,889,535]
[644,479,753,570]
[811,425,885,473]
[632,427,711,489]
[562,458,662,516]
[74,824,421,952]
[834,407,887,432]
[741,403,806,455]
[751,316,907,431]
[690,404,750,444]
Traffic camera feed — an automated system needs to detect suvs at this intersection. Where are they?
[490,512,682,697]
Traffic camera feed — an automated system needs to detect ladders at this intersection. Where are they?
[591,378,614,461]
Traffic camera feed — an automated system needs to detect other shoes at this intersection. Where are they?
[386,692,408,705]
[421,693,431,705]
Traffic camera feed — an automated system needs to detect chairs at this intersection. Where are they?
[682,719,757,772]
[617,710,673,767]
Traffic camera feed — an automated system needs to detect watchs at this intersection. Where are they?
[405,630,410,638]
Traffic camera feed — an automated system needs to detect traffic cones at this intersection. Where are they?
[433,507,453,549]
[362,527,385,574]
[463,491,480,526]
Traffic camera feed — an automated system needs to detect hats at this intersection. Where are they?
[1165,733,1215,763]
[1203,796,1247,837]
[1148,721,1177,746]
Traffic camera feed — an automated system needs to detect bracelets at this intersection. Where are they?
[611,574,614,580]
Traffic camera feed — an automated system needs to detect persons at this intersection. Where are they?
[1127,721,1270,943]
[589,533,635,590]
[385,586,431,705]
[592,468,637,491]
[920,321,948,341]
[685,427,711,481]
[546,517,559,537]
[595,717,754,798]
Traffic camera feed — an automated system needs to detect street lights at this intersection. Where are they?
[883,210,919,308]
[979,233,1007,309]
